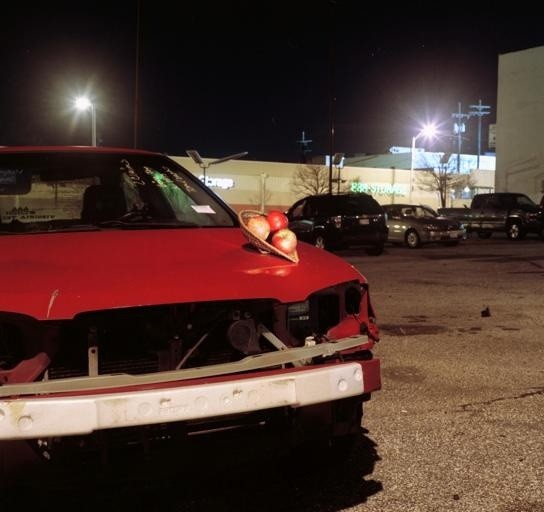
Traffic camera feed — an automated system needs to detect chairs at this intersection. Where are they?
[80,184,127,224]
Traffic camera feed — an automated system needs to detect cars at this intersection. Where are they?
[378,203,467,250]
[1,141,386,510]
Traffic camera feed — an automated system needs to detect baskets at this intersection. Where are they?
[238,208,300,263]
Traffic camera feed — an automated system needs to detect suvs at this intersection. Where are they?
[277,190,391,257]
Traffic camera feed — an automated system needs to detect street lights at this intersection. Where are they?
[75,98,100,148]
[333,150,377,194]
[439,151,451,208]
[185,146,249,189]
[409,122,438,202]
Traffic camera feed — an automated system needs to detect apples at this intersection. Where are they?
[247,211,299,254]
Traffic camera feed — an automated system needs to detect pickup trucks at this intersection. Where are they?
[436,190,544,240]
